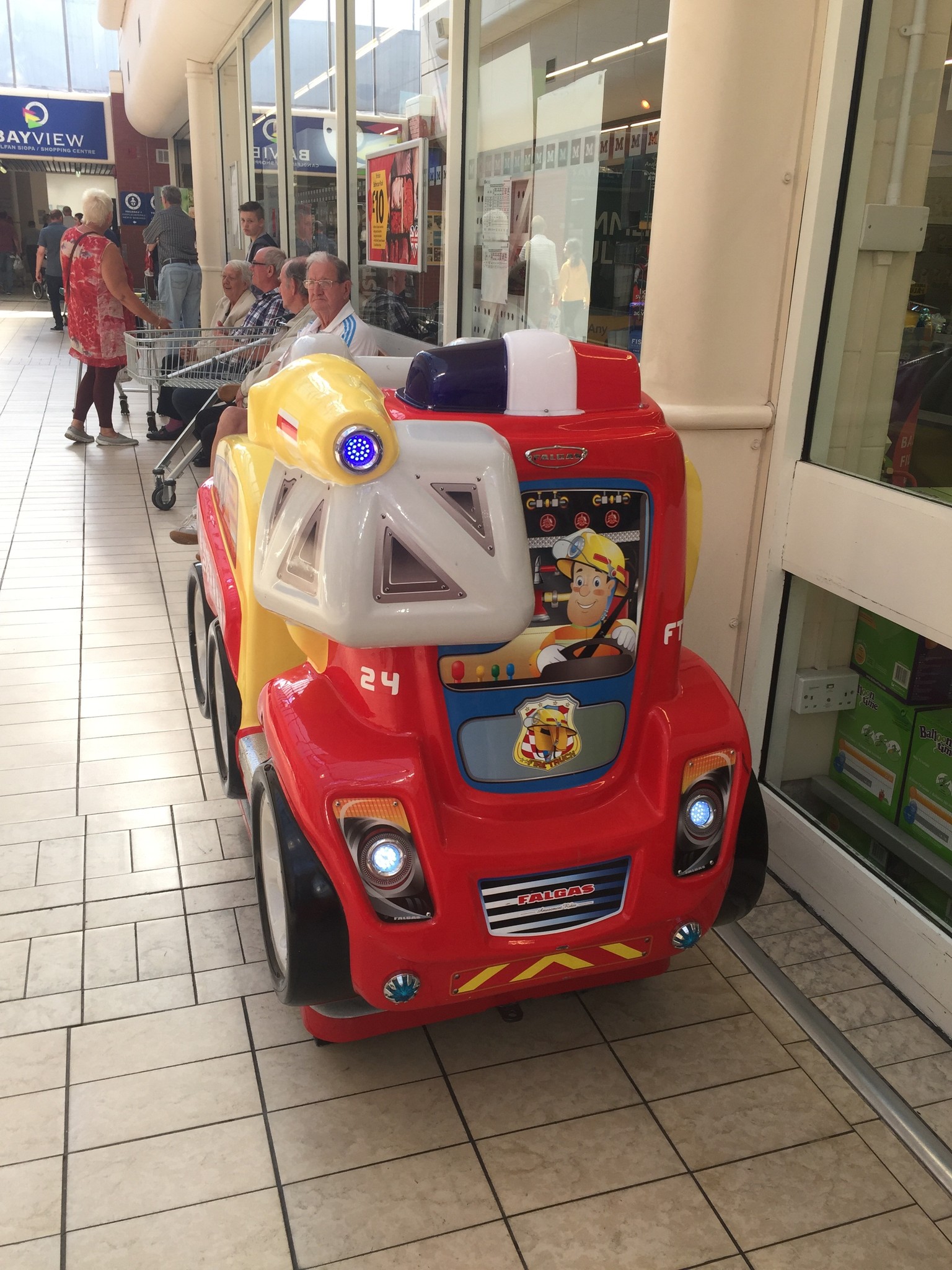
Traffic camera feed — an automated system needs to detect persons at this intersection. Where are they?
[513,216,558,330]
[59,189,174,446]
[0,205,123,333]
[294,203,315,254]
[312,221,336,251]
[363,266,440,340]
[147,238,159,289]
[146,199,380,563]
[553,237,590,337]
[143,185,202,355]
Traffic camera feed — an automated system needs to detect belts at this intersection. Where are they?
[162,259,198,267]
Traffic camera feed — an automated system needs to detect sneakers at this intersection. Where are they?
[50,326,64,332]
[196,552,201,561]
[169,505,198,545]
[65,426,94,442]
[95,432,139,446]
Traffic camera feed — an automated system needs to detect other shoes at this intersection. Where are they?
[194,457,210,467]
[192,452,204,461]
[6,291,12,294]
[146,426,184,440]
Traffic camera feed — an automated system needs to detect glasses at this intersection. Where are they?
[303,279,344,290]
[251,261,272,267]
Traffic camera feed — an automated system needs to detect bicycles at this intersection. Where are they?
[32,254,51,301]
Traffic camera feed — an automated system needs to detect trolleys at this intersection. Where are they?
[124,312,296,511]
[58,287,161,415]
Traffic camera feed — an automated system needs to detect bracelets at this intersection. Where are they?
[155,316,161,329]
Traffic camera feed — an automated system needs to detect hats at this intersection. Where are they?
[28,221,35,225]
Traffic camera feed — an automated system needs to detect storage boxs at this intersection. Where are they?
[828,485,952,932]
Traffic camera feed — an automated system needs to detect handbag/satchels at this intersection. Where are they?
[144,244,155,277]
[9,253,26,275]
